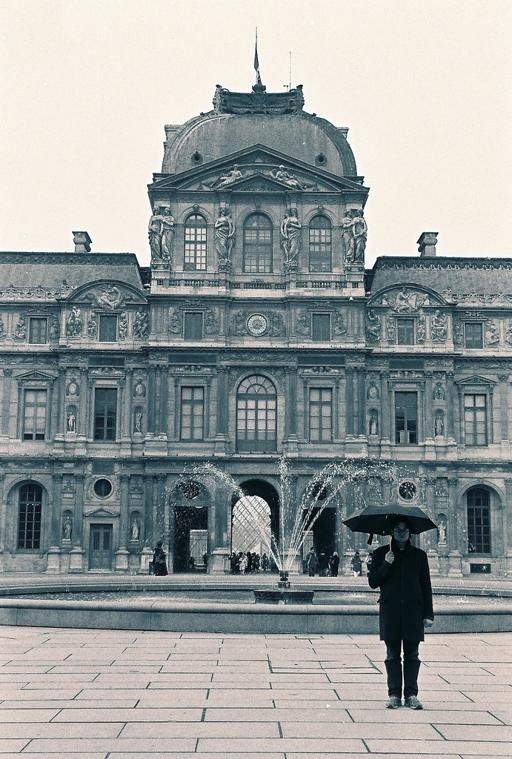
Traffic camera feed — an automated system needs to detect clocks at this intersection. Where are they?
[246,313,270,337]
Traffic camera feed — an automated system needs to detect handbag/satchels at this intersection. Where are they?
[156,555,165,563]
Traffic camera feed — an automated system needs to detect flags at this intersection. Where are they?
[253,45,259,71]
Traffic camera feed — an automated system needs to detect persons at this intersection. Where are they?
[329,552,339,577]
[318,550,329,577]
[228,550,271,575]
[148,207,368,272]
[63,516,72,539]
[366,287,448,347]
[135,412,142,432]
[66,286,150,342]
[67,412,76,431]
[202,552,207,563]
[436,520,446,544]
[132,518,140,540]
[214,162,243,190]
[306,546,318,577]
[366,518,435,711]
[369,414,377,435]
[434,416,444,435]
[275,165,307,189]
[350,552,364,577]
[153,541,168,577]
[364,553,372,576]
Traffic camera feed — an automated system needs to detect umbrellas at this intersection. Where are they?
[342,502,438,551]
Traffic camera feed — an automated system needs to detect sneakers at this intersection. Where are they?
[404,696,422,709]
[385,696,402,708]
